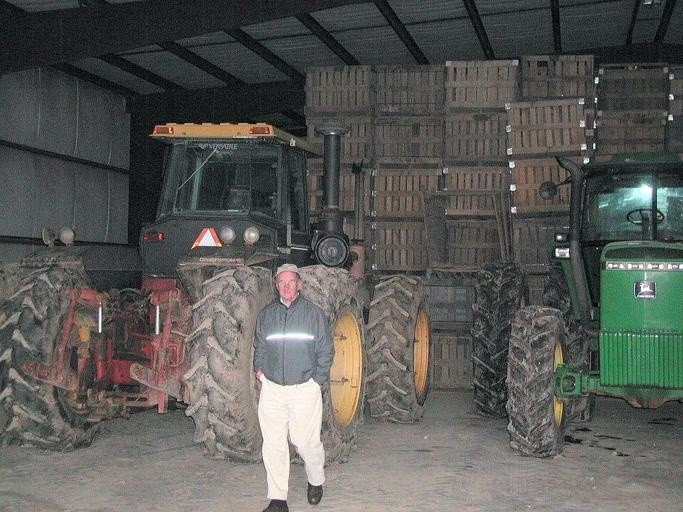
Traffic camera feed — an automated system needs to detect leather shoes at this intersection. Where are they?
[262,499,289,512]
[307,481,324,505]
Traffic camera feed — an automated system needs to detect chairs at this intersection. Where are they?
[217,184,260,214]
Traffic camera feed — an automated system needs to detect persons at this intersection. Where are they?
[253,262,335,512]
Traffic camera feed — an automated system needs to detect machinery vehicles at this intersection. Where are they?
[468,147,681,458]
[0,119,434,464]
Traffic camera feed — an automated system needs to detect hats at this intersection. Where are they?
[274,262,301,278]
